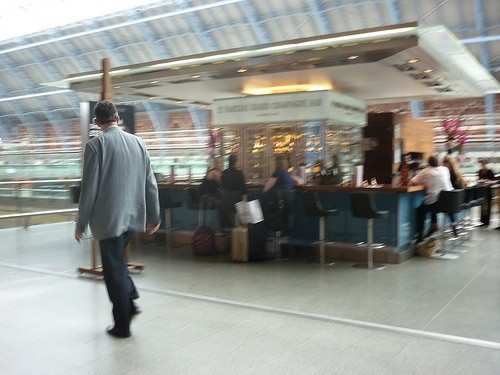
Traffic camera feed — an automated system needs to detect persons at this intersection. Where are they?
[476,159,496,228]
[72,100,162,338]
[252,156,303,238]
[409,156,454,245]
[396,154,416,186]
[438,154,467,238]
[220,154,248,213]
[199,156,235,231]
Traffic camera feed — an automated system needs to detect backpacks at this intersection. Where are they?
[193,225,216,255]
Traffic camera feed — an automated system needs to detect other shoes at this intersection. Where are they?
[107,324,131,339]
[416,238,423,244]
[129,300,138,320]
[425,224,438,237]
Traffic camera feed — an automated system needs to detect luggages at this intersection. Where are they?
[231,225,249,263]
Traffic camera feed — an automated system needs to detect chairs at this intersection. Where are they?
[187,187,218,227]
[300,191,341,266]
[430,189,465,260]
[69,186,92,239]
[158,188,184,250]
[262,190,298,263]
[349,192,390,271]
[450,185,491,252]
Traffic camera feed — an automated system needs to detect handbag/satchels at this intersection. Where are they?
[416,237,438,256]
[234,195,264,225]
